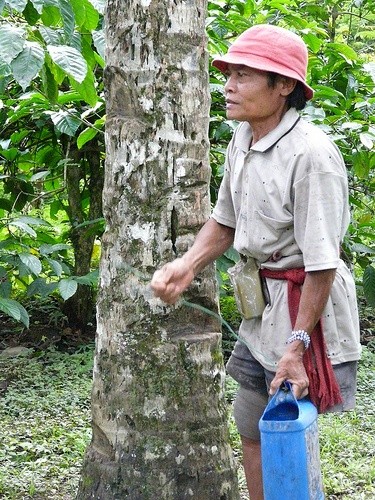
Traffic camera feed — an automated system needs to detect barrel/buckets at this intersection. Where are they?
[257,379,324,499]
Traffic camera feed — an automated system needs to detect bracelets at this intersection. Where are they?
[285,330,311,353]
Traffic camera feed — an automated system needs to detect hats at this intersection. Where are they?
[212,23,314,101]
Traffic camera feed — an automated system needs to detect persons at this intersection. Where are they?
[146,22,363,500]
